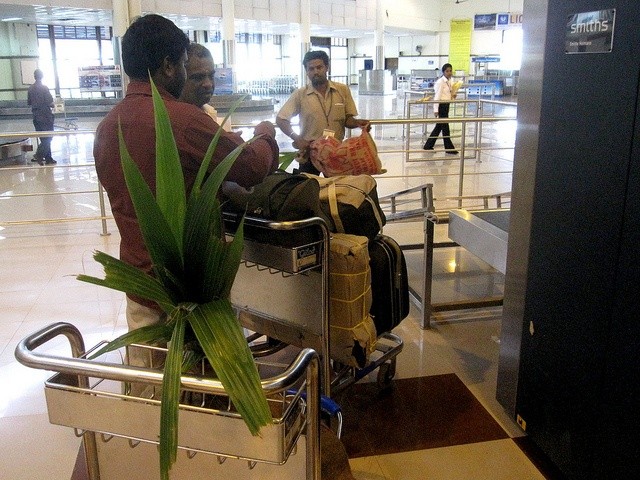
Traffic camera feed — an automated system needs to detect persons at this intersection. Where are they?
[175,42,217,126]
[423,63,459,154]
[275,50,372,179]
[91,12,280,402]
[26,68,57,165]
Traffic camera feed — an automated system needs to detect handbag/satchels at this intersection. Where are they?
[367,234,410,338]
[292,169,386,244]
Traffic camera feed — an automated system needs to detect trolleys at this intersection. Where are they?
[14,321,343,480]
[222,212,405,397]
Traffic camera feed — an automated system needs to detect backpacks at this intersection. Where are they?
[256,171,335,243]
